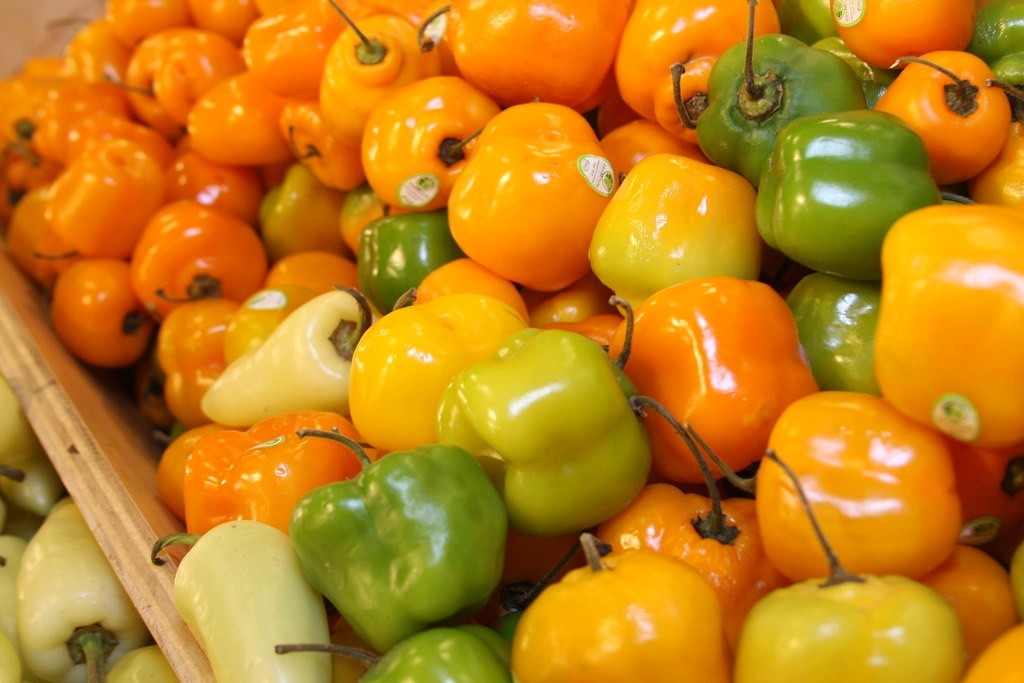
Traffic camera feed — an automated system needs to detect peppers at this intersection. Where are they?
[0,0,1024,683]
